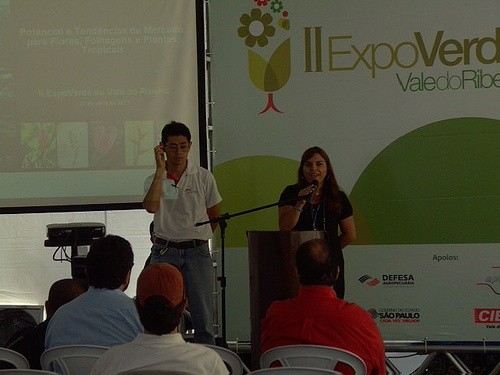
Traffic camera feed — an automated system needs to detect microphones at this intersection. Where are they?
[308,180,319,202]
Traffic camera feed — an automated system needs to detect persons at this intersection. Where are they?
[143,122,224,345]
[277,146,357,300]
[0,220,385,375]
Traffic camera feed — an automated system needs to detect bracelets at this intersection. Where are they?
[293,206,303,214]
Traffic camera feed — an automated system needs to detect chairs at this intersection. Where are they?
[0,345,367,375]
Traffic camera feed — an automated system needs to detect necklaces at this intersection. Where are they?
[315,192,320,196]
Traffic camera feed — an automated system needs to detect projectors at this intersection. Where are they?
[46,223,106,240]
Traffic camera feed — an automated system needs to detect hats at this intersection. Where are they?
[137,263,188,310]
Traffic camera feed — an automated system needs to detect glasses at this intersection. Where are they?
[165,144,190,153]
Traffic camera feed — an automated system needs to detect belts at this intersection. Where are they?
[156,237,208,249]
